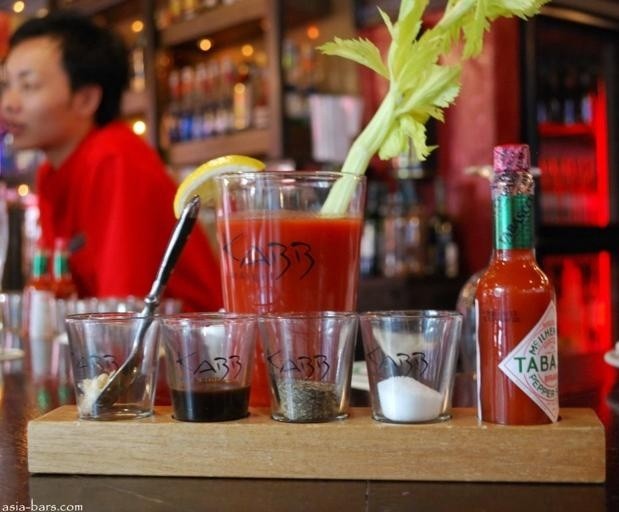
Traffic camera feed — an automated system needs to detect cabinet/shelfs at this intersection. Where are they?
[145,1,327,188]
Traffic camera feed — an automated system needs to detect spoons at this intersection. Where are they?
[92,193,202,421]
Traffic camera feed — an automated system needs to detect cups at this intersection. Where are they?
[216,168,366,419]
[63,310,466,425]
[1,293,181,358]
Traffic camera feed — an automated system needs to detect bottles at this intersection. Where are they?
[473,144,564,428]
[374,145,463,283]
[230,68,252,129]
[28,345,73,410]
[23,237,79,344]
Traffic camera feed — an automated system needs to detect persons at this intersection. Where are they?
[1,9,224,312]
